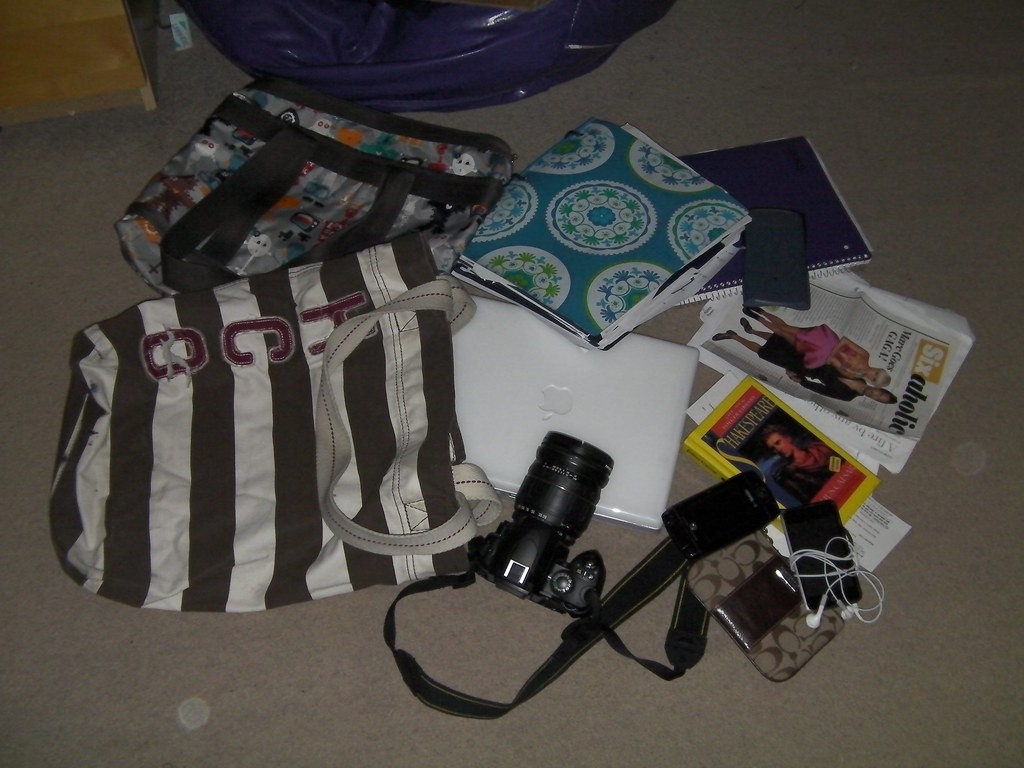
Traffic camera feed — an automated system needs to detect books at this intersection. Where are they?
[674,134,873,308]
[682,373,882,537]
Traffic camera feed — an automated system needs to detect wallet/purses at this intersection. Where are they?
[687,530,844,682]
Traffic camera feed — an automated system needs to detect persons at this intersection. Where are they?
[712,318,898,406]
[761,424,845,504]
[742,304,891,386]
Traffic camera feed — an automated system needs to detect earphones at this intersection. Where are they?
[837,599,859,620]
[806,594,828,629]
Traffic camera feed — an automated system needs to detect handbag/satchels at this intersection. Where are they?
[46,231,472,614]
[176,0,679,113]
[115,73,517,293]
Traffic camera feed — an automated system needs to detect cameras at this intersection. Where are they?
[465,430,614,620]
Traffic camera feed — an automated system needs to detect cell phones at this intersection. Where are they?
[744,208,811,311]
[661,471,779,561]
[779,499,863,610]
